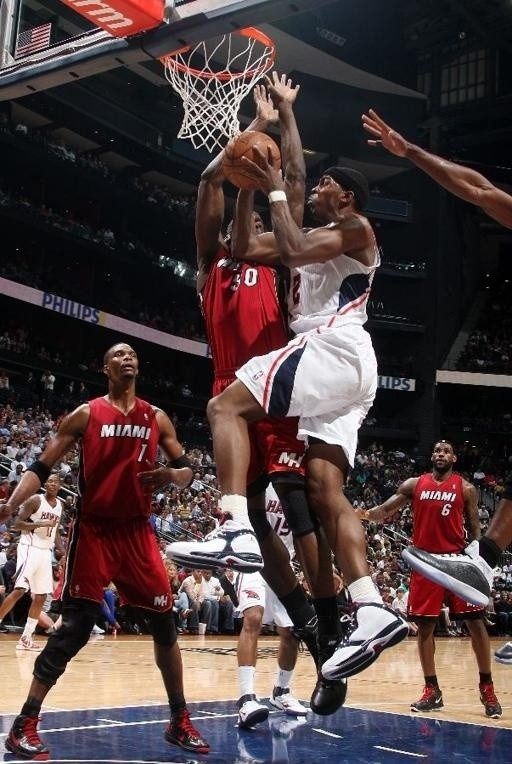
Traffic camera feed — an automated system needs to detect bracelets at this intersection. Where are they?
[266,190,288,203]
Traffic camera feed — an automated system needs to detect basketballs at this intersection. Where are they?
[223,131,281,191]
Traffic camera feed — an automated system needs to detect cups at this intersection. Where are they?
[198,623,208,635]
[112,625,116,635]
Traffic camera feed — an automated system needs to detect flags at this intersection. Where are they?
[14,22,51,58]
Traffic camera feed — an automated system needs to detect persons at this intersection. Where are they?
[163,145,407,684]
[1,399,512,635]
[359,108,512,667]
[351,440,503,719]
[1,343,209,759]
[193,67,347,715]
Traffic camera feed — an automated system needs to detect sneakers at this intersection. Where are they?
[269,687,307,716]
[16,638,45,653]
[236,702,269,728]
[163,714,211,755]
[166,511,264,574]
[4,712,51,762]
[493,640,512,664]
[235,726,270,763]
[477,683,503,719]
[401,539,493,607]
[322,604,410,682]
[92,625,188,635]
[163,745,207,764]
[289,613,352,715]
[269,715,308,741]
[409,688,445,713]
